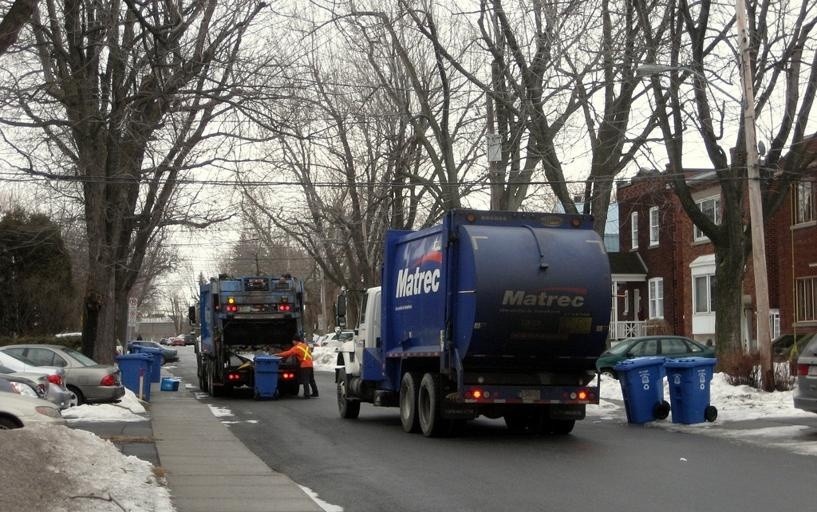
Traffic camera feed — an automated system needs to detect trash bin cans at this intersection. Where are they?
[612,356,720,424]
[253,356,283,400]
[113,346,161,402]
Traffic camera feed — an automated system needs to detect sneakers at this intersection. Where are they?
[310,392,319,398]
[298,394,310,400]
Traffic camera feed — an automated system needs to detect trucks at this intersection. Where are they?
[189,272,305,397]
[334,207,614,437]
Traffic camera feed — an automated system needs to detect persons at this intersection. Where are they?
[271,334,319,401]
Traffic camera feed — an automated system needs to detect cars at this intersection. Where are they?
[0,344,125,430]
[127,340,179,366]
[313,332,355,352]
[772,332,817,414]
[596,335,721,380]
[159,333,196,346]
